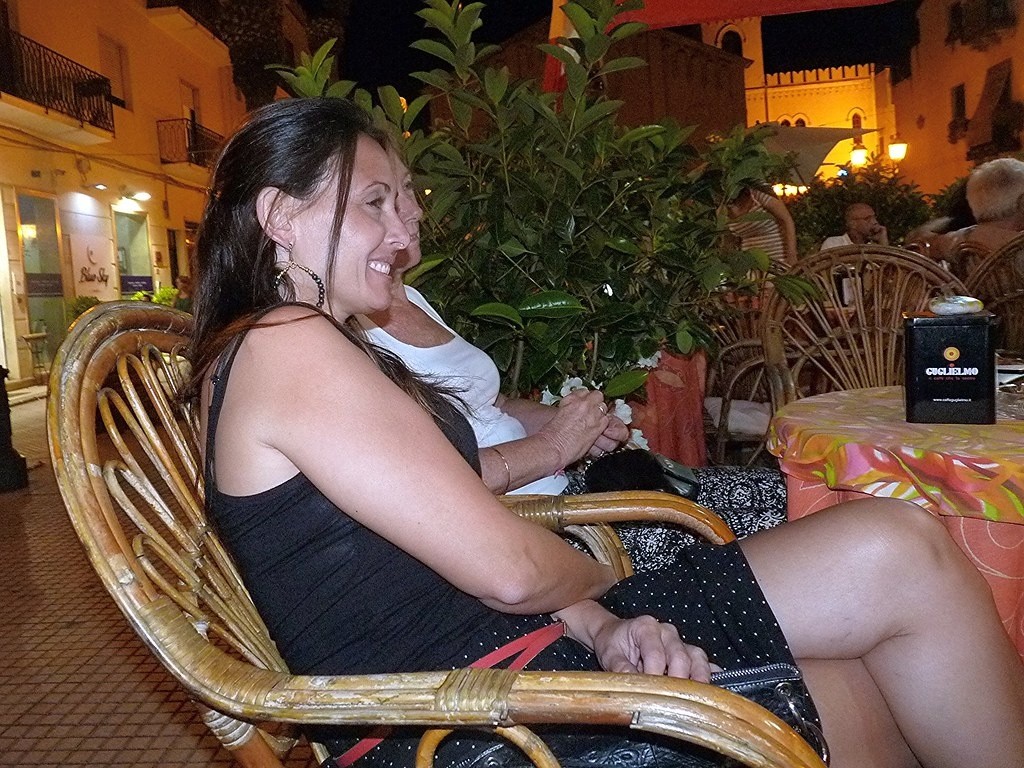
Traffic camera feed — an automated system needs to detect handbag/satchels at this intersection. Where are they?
[447,662,830,768]
[589,447,700,500]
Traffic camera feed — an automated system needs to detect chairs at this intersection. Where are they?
[689,186,1024,472]
[46,298,828,768]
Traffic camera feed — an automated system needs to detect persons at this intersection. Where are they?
[141,294,151,303]
[173,96,1024,768]
[172,275,194,316]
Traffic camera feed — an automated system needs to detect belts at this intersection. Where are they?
[331,619,567,768]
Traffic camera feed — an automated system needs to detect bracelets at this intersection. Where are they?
[491,447,511,495]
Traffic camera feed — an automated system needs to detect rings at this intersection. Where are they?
[596,403,606,416]
[597,450,604,459]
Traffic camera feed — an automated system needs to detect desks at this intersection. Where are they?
[760,382,1024,668]
